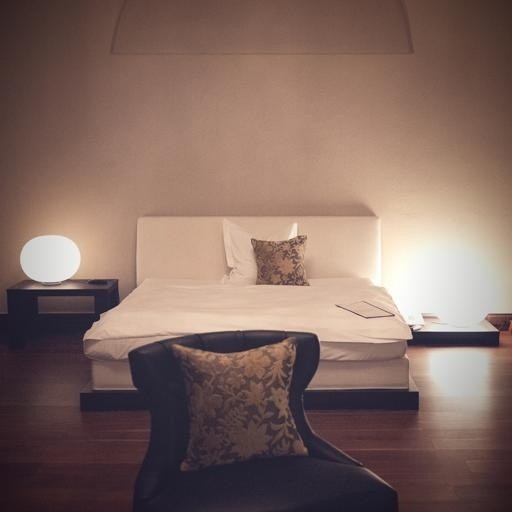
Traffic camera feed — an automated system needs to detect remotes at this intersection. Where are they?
[89,278,108,284]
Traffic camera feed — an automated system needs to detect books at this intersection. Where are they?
[335,299,395,319]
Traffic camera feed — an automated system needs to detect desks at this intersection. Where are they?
[5,279,119,350]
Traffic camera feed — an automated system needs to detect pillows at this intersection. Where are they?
[218,218,311,288]
[169,334,311,476]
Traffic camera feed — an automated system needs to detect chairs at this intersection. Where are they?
[128,327,400,511]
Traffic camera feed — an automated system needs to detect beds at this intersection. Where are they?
[77,213,422,412]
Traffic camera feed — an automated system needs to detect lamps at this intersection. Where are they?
[21,234,81,287]
